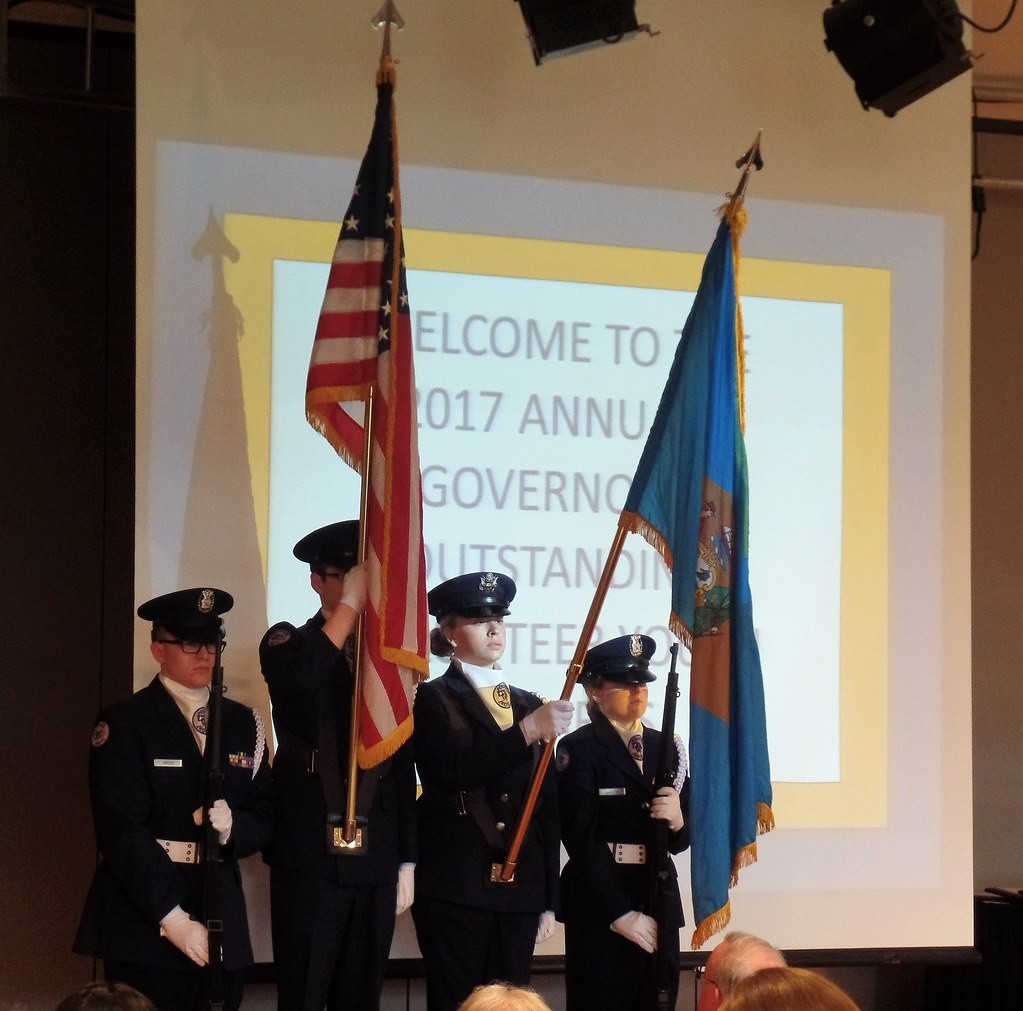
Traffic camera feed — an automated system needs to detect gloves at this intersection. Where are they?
[650,787,684,833]
[609,912,659,954]
[396,863,416,914]
[536,909,555,944]
[209,802,232,845]
[522,697,573,743]
[160,904,223,965]
[339,559,372,615]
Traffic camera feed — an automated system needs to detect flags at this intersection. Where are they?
[300,56,428,776]
[612,198,782,934]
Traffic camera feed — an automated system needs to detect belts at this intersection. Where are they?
[155,837,222,864]
[608,843,652,864]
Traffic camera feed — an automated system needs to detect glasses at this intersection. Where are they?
[694,965,716,985]
[318,572,344,583]
[152,635,227,655]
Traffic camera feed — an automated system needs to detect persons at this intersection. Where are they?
[59,929,861,1011]
[555,635,692,1011]
[413,572,573,1011]
[262,519,419,1010]
[71,587,288,1010]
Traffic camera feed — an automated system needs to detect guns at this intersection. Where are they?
[656,639,679,1011]
[200,630,228,1010]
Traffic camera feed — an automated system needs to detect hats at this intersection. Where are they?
[426,572,516,617]
[138,585,234,641]
[566,634,657,686]
[292,520,361,570]
